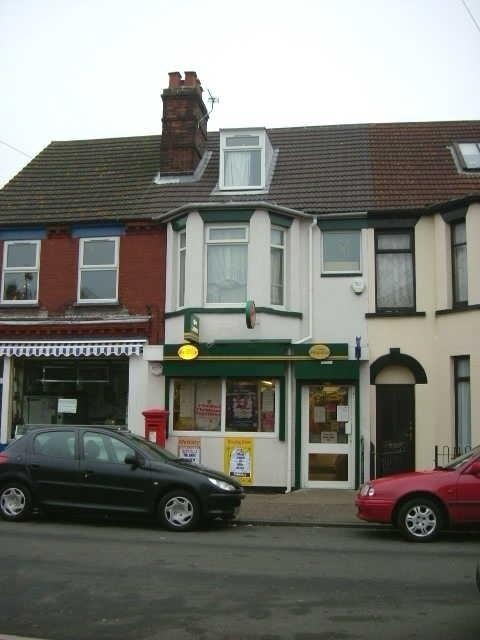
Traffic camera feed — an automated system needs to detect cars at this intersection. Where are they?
[1,425,245,532]
[371,426,411,476]
[355,443,479,544]
